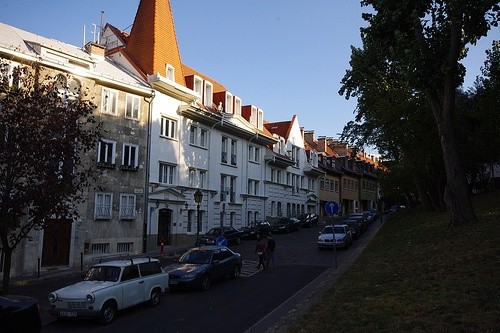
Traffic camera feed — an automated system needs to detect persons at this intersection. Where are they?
[255,234,276,270]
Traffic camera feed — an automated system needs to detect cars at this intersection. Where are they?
[340,205,406,238]
[241,219,271,237]
[163,245,243,292]
[0,293,43,333]
[317,224,356,250]
[297,213,319,227]
[270,217,302,234]
[198,225,249,246]
[46,256,172,325]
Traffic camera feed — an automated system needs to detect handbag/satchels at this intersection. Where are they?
[257,251,264,258]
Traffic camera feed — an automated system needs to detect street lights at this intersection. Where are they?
[193,187,203,247]
